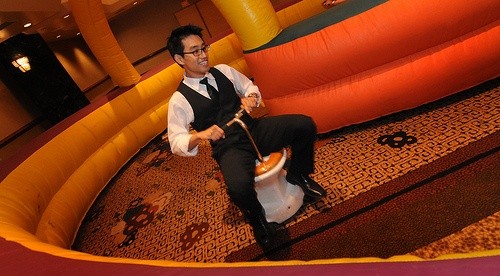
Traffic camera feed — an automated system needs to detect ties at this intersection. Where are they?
[199,77,218,102]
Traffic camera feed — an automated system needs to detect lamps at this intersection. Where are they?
[3,41,32,73]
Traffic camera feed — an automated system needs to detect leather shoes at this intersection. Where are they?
[252,215,275,247]
[285,171,327,199]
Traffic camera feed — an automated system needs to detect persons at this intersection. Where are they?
[165,25,326,245]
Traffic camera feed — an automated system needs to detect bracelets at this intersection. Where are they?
[248,93,257,98]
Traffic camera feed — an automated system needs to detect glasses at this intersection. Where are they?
[182,43,210,57]
